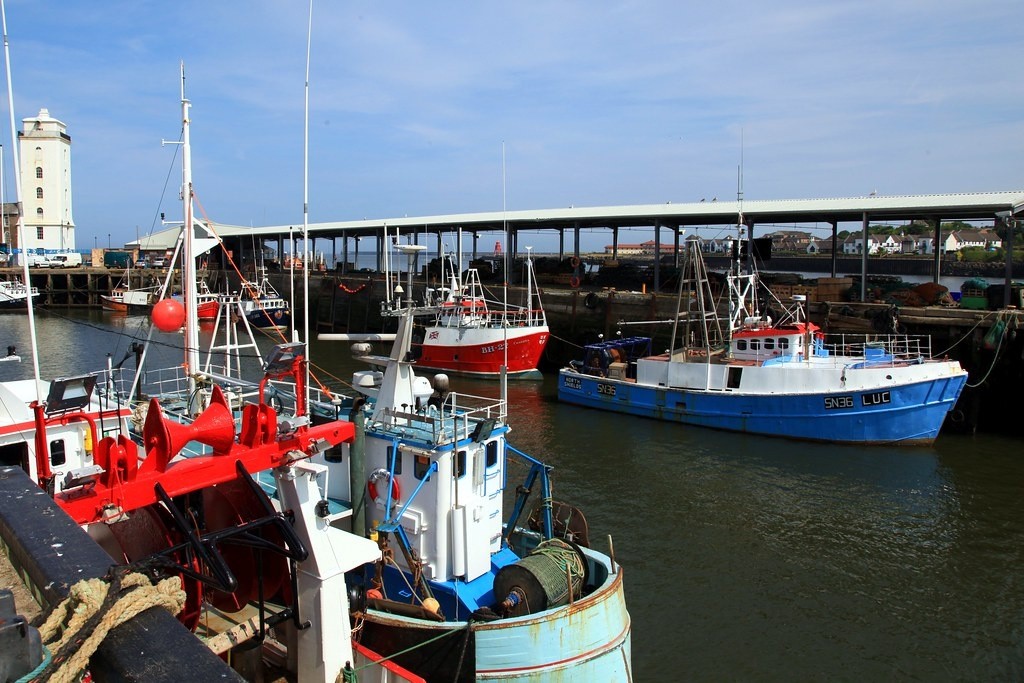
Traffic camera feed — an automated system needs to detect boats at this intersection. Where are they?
[0,275,40,308]
[407,227,550,378]
[1,2,429,682]
[100,259,162,311]
[92,2,634,682]
[171,275,220,321]
[221,218,291,323]
[556,165,969,447]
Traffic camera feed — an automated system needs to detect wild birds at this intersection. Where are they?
[712,197,718,202]
[667,200,672,204]
[569,206,573,208]
[363,217,367,220]
[404,214,407,217]
[700,198,706,202]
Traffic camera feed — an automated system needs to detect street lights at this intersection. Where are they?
[94,236,97,248]
[108,233,111,249]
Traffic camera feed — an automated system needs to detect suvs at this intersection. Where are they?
[135,259,148,268]
[151,257,170,268]
[27,255,50,267]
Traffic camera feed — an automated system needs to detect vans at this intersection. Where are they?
[104,251,134,268]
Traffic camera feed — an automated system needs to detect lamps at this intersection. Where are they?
[58,464,107,491]
[44,374,98,416]
[262,342,306,371]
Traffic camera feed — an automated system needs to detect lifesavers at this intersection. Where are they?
[368,467,400,512]
[570,255,579,269]
[569,276,581,288]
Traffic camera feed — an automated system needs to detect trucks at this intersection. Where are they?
[49,252,82,268]
